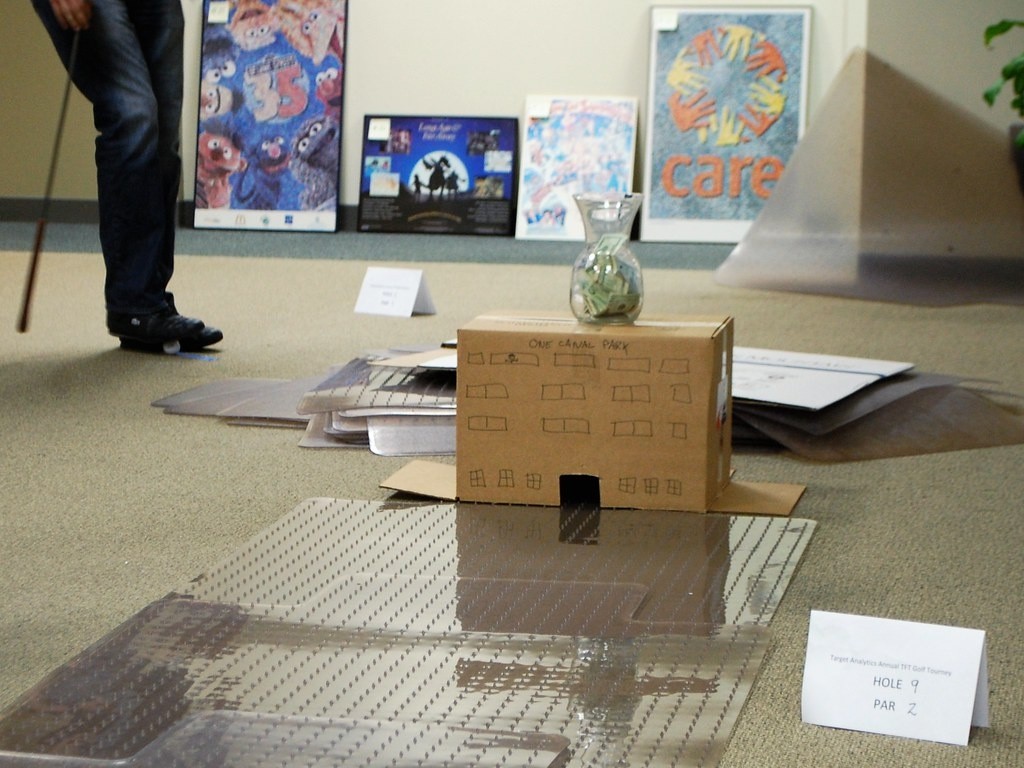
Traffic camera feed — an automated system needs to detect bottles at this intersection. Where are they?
[569,188,648,326]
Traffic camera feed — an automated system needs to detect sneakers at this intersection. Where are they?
[106,301,205,343]
[119,323,222,351]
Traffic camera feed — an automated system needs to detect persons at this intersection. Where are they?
[28,0,224,349]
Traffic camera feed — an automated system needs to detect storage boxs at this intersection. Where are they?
[377,306,808,517]
